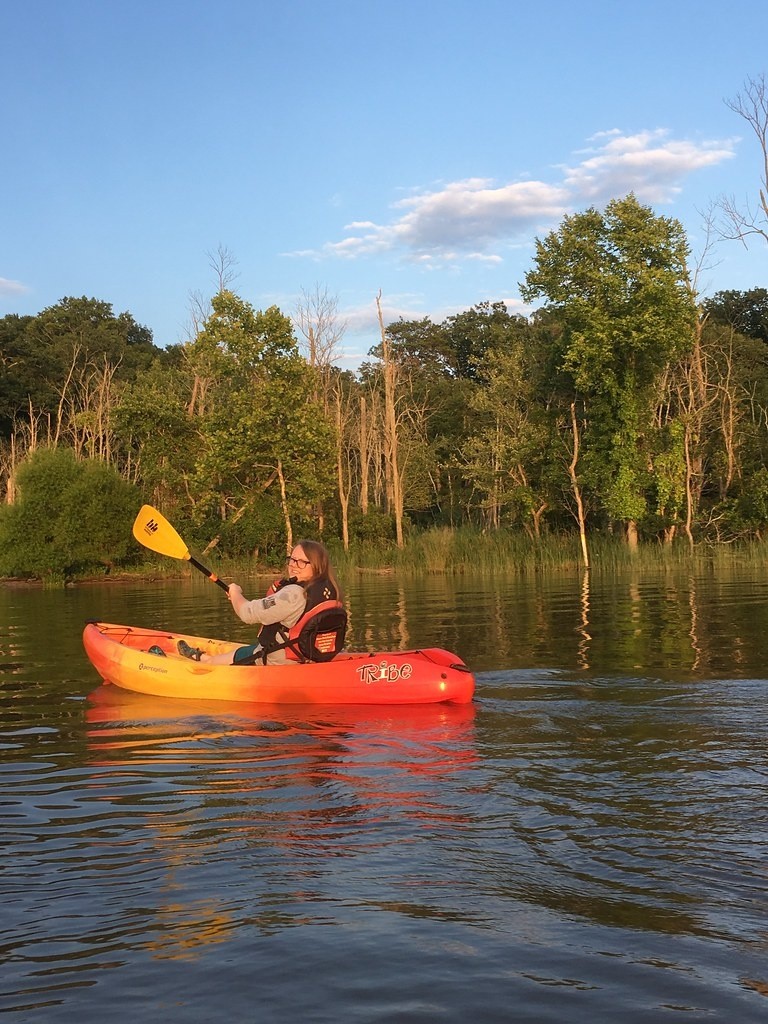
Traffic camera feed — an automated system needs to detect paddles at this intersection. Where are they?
[133,502,231,592]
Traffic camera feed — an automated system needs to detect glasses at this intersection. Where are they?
[285,556,310,569]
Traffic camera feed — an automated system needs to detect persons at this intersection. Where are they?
[148,540,348,666]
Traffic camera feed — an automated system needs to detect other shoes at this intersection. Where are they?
[177,640,206,661]
[148,645,166,657]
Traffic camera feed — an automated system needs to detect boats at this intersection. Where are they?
[84,619,472,704]
